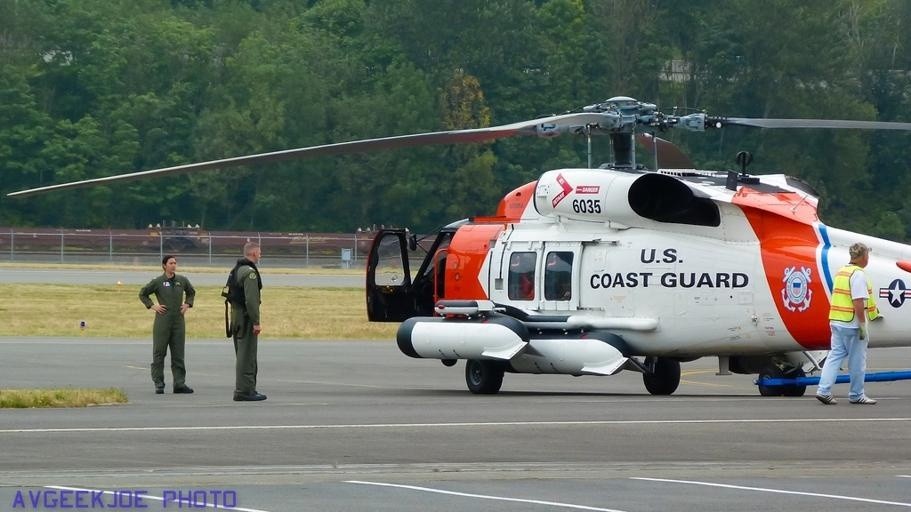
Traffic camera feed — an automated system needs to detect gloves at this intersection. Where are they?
[858,320,866,340]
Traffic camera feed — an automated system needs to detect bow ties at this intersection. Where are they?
[848,396,877,404]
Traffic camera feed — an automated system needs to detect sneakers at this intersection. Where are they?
[814,391,837,404]
[233,390,268,401]
[155,385,165,394]
[172,385,194,393]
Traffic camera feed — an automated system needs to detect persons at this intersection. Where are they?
[139,255,195,394]
[221,242,266,401]
[816,242,879,404]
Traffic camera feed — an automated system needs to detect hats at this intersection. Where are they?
[848,242,873,260]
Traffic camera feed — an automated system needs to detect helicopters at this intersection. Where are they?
[7,95,911,398]
